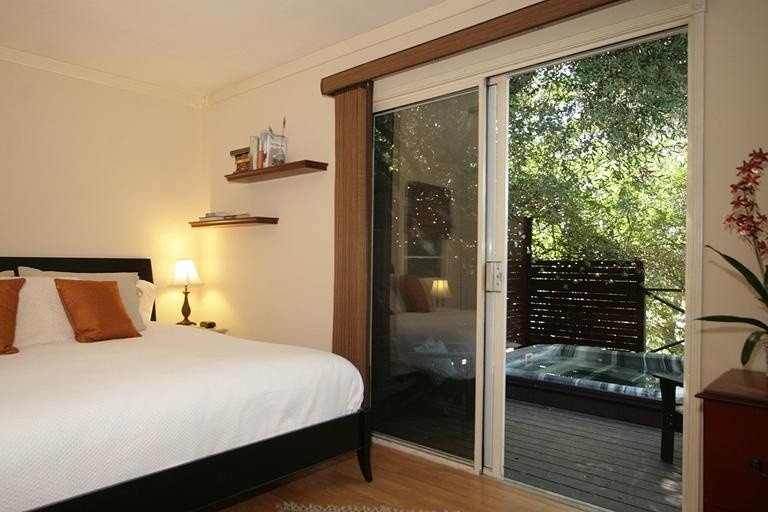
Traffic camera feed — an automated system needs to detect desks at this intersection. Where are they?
[653,371,683,466]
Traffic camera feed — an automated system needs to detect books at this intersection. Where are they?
[199,213,251,222]
[204,210,247,218]
[228,130,286,175]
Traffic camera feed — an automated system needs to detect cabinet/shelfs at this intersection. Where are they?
[695,368,768,512]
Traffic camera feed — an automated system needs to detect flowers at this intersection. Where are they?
[694,146,768,372]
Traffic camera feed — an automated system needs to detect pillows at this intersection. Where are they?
[0,266,158,357]
[390,273,430,314]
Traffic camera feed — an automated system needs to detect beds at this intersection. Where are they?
[385,273,477,382]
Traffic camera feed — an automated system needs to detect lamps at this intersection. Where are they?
[430,279,451,307]
[168,258,204,325]
